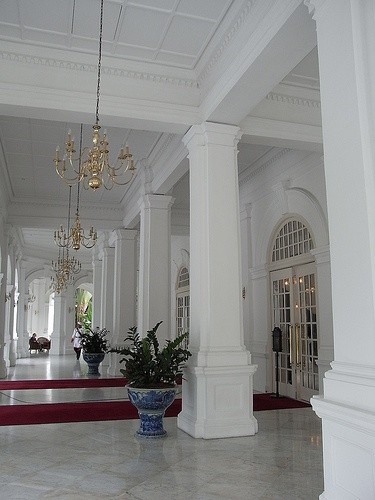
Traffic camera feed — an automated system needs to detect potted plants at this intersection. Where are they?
[76,325,110,375]
[107,320,192,441]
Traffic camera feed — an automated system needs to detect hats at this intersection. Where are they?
[78,321,83,326]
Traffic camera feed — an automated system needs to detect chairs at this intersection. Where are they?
[29,337,50,355]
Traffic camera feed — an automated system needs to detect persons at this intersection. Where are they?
[70,322,84,359]
[29,333,44,352]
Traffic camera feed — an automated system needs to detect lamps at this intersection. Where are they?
[48,0,138,295]
